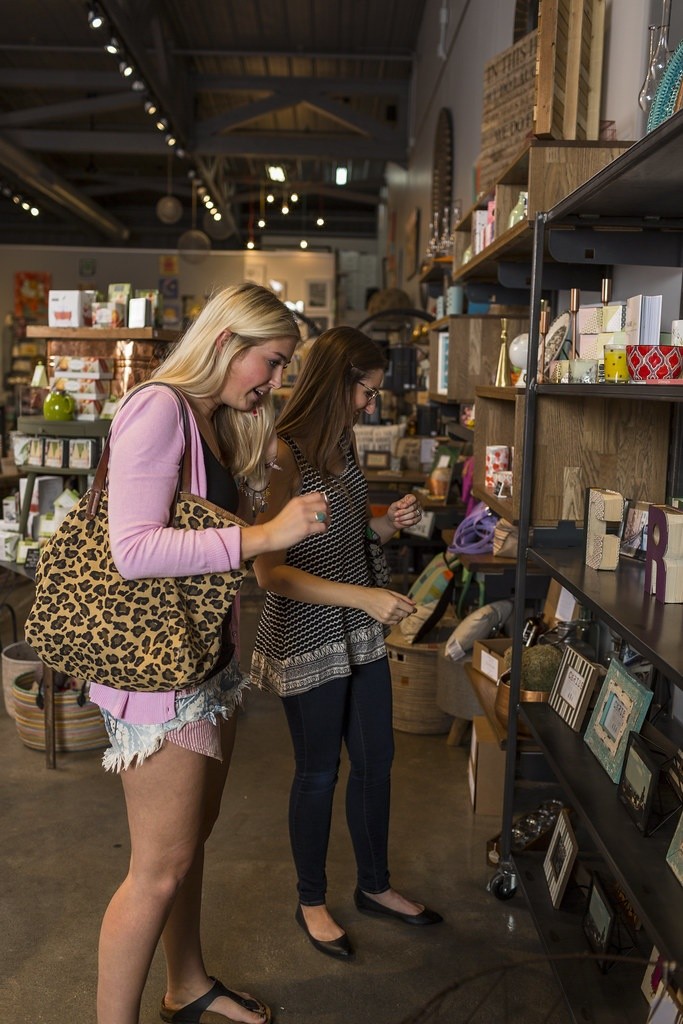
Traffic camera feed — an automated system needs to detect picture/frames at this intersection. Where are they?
[542,809,578,908]
[584,658,654,783]
[302,312,335,342]
[548,644,598,731]
[616,730,659,835]
[582,870,615,974]
[301,278,332,314]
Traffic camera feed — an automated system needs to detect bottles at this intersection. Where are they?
[489,855,516,901]
[43,390,73,422]
[573,632,595,661]
[490,622,505,639]
[521,612,545,645]
[507,191,525,229]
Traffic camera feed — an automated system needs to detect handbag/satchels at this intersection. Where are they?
[23,381,255,694]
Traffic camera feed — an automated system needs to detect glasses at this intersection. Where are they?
[356,380,381,404]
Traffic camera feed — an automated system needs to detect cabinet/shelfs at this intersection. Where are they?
[503,112,683,1023]
[430,316,527,400]
[453,139,637,288]
[460,386,669,750]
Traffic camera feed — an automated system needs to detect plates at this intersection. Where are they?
[537,311,572,381]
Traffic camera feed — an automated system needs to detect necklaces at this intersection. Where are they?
[200,413,221,463]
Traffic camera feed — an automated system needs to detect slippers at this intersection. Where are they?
[160,977,271,1024]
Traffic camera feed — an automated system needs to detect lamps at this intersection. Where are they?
[87,9,235,263]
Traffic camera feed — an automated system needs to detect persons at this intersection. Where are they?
[252,326,444,959]
[87,278,332,1024]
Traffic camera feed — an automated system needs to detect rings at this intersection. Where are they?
[416,509,421,516]
[320,492,328,499]
[314,510,326,522]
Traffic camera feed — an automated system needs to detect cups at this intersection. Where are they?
[568,359,599,384]
[671,320,683,346]
[486,445,508,488]
[604,344,630,385]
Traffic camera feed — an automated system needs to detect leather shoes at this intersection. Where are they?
[294,903,356,960]
[352,885,445,928]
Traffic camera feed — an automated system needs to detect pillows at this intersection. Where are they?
[401,552,456,644]
[354,422,435,470]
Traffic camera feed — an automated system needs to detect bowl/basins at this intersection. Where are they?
[626,345,683,380]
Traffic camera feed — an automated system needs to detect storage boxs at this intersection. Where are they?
[0,284,185,563]
[467,715,506,814]
[472,576,581,684]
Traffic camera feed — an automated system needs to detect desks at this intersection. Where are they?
[16,464,97,541]
